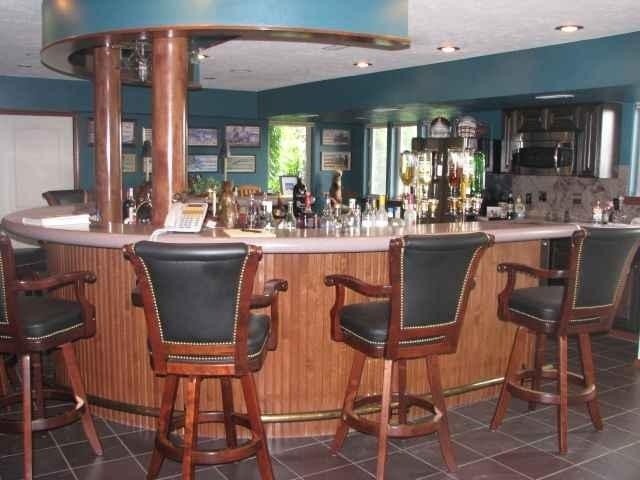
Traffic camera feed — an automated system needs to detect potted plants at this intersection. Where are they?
[187,126,218,147]
[88,117,137,147]
[320,127,352,147]
[188,154,219,174]
[320,151,352,172]
[223,125,262,149]
[227,156,257,174]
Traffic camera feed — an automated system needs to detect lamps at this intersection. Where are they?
[324,232,494,480]
[0,235,103,480]
[489,229,640,454]
[121,241,288,480]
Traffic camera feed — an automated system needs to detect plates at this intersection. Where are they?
[518,106,548,133]
[497,106,523,175]
[548,104,580,131]
[578,102,621,180]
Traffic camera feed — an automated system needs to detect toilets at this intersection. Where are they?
[148,201,208,242]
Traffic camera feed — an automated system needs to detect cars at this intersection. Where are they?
[392,219,405,234]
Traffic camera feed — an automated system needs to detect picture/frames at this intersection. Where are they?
[1,196,580,438]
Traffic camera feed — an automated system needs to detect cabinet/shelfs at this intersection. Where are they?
[343,198,360,229]
[319,197,333,228]
[292,175,308,218]
[391,206,405,226]
[362,195,371,226]
[284,201,296,230]
[515,192,525,215]
[404,194,417,224]
[375,195,388,226]
[123,187,137,224]
[592,199,603,222]
[507,191,514,220]
[333,203,342,229]
[368,198,378,226]
